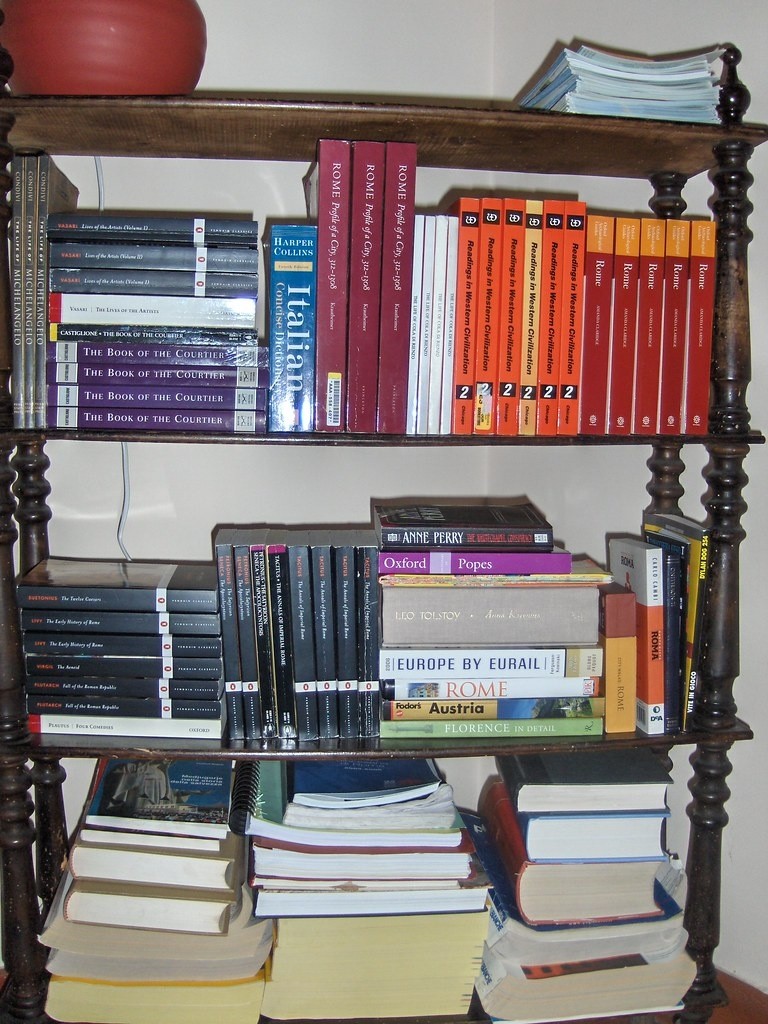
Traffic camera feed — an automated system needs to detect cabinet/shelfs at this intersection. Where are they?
[0,10,768,1024]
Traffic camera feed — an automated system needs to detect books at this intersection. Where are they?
[13,139,715,1024]
[521,45,727,125]
